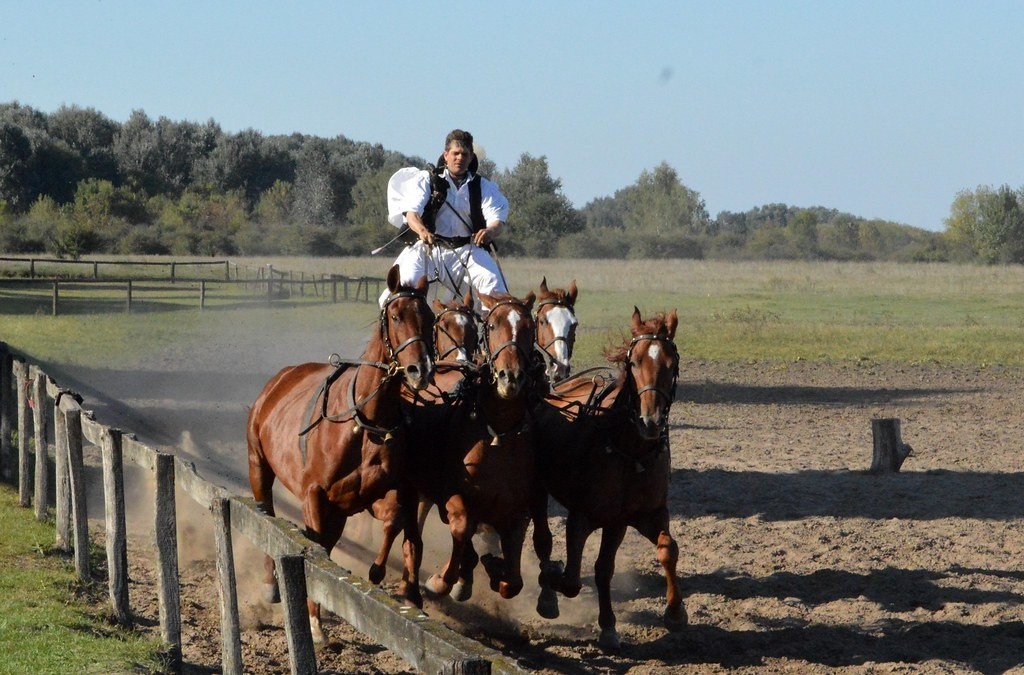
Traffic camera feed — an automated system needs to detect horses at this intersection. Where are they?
[246,264,687,647]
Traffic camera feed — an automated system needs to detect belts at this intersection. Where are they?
[433,234,471,249]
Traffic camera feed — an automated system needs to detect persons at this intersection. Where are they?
[379,129,509,314]
